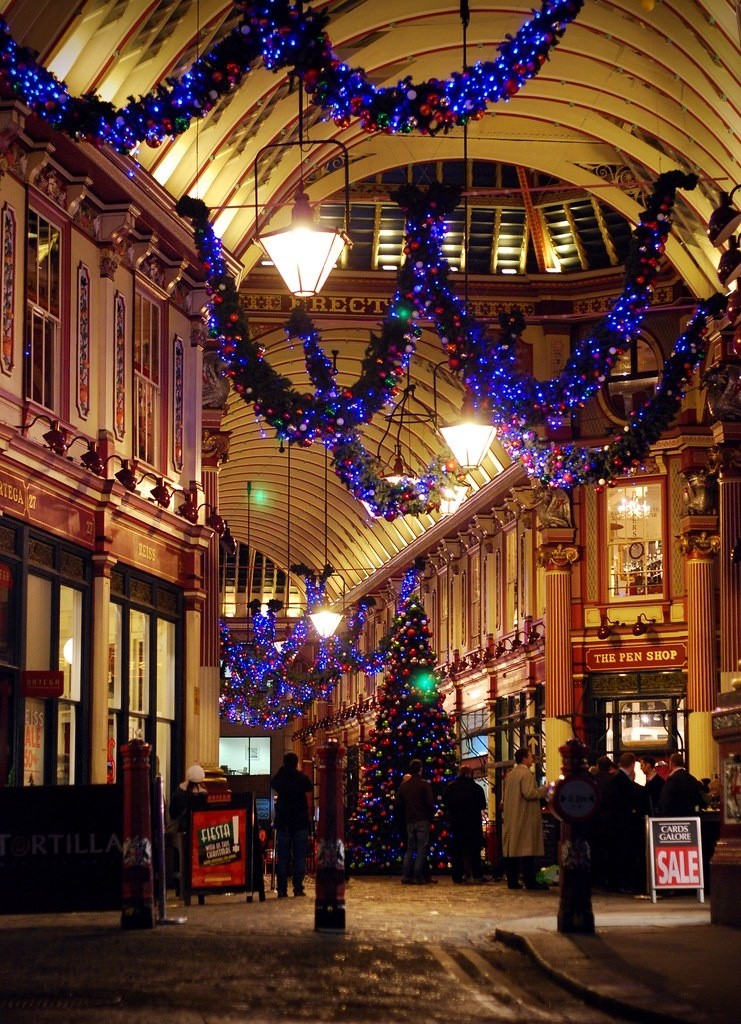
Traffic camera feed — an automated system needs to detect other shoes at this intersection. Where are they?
[403,877,438,885]
[279,891,306,897]
[508,883,550,891]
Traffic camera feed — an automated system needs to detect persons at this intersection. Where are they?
[580,742,719,903]
[500,749,551,890]
[442,765,485,880]
[397,755,436,885]
[268,754,313,900]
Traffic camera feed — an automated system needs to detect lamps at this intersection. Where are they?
[436,397,500,475]
[437,477,470,517]
[100,454,138,493]
[291,622,545,741]
[632,612,656,636]
[219,517,237,555]
[13,414,69,457]
[136,472,171,509]
[598,615,620,640]
[175,501,226,535]
[307,596,346,638]
[255,195,349,298]
[149,488,198,525]
[42,435,103,477]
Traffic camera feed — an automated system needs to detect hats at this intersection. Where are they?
[186,766,205,782]
